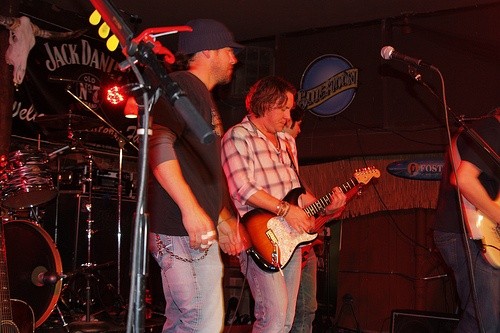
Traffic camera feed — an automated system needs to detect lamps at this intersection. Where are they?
[123,97,140,119]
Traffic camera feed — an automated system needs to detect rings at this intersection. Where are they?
[211,230,216,235]
[208,240,213,245]
[207,231,212,237]
[201,235,207,239]
[212,239,216,243]
[200,244,207,248]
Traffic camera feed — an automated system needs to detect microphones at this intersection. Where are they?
[391,58,421,81]
[380,46,438,71]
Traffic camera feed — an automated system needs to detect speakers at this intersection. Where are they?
[390,309,460,333]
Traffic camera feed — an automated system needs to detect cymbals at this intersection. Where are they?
[47,77,85,84]
[35,112,100,130]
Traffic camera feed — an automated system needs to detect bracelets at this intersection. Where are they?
[218,215,235,224]
[276,200,290,218]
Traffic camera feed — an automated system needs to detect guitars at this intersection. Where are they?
[0,206,36,333]
[238,166,380,273]
[474,188,500,271]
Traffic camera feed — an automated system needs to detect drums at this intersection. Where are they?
[0,219,64,330]
[0,147,59,211]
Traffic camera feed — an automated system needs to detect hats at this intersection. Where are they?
[177,19,245,56]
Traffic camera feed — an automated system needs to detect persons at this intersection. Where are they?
[220,74,347,333]
[435,106,500,333]
[141,19,242,333]
[282,104,317,333]
